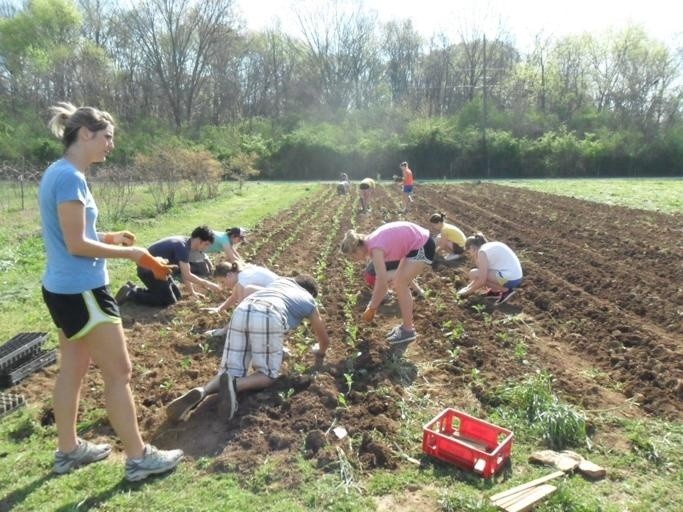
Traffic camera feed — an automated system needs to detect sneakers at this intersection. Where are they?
[53,438,112,475]
[480,288,515,305]
[126,443,185,483]
[218,373,239,425]
[386,325,418,344]
[166,388,203,424]
[115,281,135,305]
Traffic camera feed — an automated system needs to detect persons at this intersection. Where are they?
[340,174,347,181]
[38,102,185,482]
[360,178,375,213]
[337,179,352,196]
[165,273,329,424]
[456,233,523,305]
[189,228,247,274]
[198,262,281,338]
[341,210,468,345]
[115,225,223,306]
[398,162,414,212]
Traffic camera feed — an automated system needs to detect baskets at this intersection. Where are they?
[419,407,513,479]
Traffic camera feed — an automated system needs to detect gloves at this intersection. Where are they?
[363,306,377,323]
[135,250,178,282]
[104,230,137,247]
